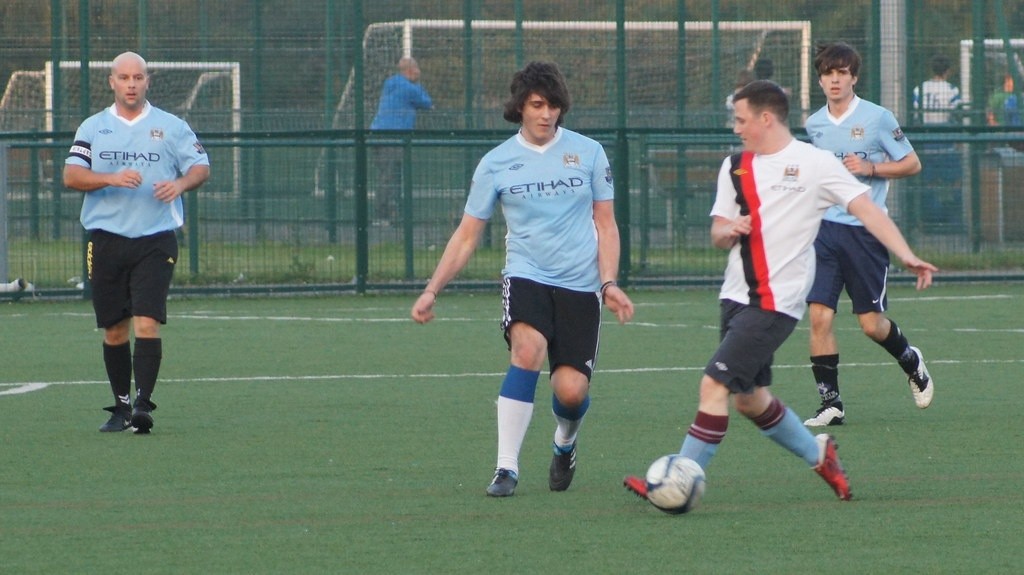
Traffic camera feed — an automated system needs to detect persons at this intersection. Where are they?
[623,81,938,501]
[62,51,211,435]
[411,62,634,496]
[368,57,433,226]
[725,59,793,128]
[803,42,935,427]
[913,56,1024,232]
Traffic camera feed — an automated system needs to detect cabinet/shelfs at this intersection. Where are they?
[980,150,1024,246]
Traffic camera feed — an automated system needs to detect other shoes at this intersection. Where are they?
[372,219,390,227]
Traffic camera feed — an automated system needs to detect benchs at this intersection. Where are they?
[647,149,734,253]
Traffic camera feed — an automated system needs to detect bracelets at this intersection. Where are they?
[600,281,617,304]
[419,290,437,299]
[872,165,875,177]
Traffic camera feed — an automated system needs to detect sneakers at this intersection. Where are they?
[132,397,156,434]
[803,405,846,426]
[486,467,518,496]
[810,434,854,501]
[905,346,934,410]
[549,438,577,491]
[623,475,648,500]
[99,406,133,432]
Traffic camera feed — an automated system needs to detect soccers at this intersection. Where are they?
[644,454,708,515]
